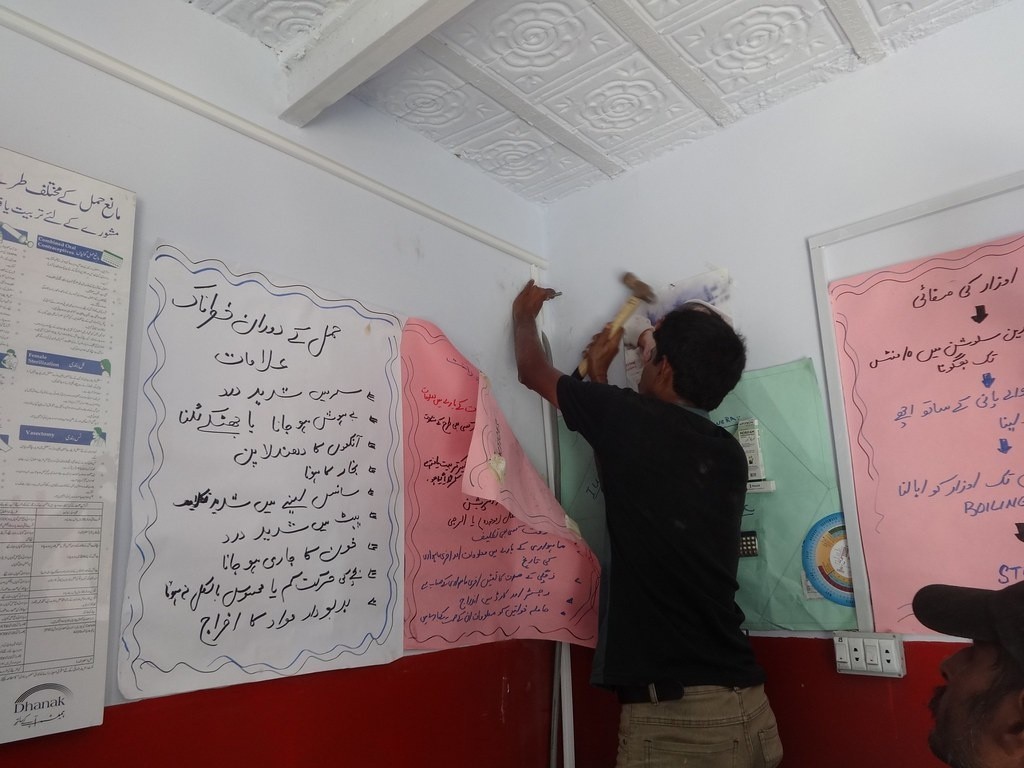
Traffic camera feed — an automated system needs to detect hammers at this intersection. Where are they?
[566,271,660,380]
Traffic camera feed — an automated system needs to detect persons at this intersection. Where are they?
[911,577,1024,767]
[512,279,783,768]
[621,312,657,363]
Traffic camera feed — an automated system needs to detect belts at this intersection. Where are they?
[616,675,685,706]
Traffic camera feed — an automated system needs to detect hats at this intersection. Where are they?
[912,581,1024,659]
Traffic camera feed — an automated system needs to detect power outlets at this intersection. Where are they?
[878,639,899,674]
[848,639,867,671]
